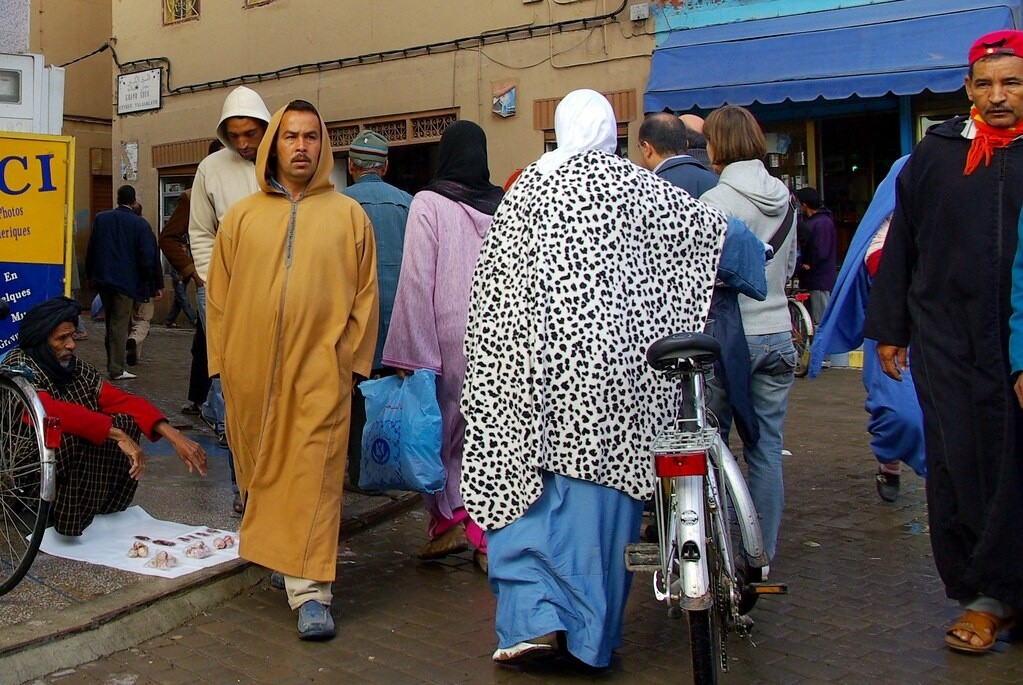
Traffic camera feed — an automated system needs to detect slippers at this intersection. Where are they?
[943,606,1016,653]
[490,641,555,664]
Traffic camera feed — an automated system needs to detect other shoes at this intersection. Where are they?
[732,554,770,582]
[294,601,337,640]
[180,402,202,415]
[819,360,832,368]
[874,464,902,504]
[112,370,139,380]
[165,322,182,329]
[412,528,470,561]
[472,549,489,575]
[71,332,90,340]
[124,338,137,367]
[269,569,287,590]
[232,492,244,513]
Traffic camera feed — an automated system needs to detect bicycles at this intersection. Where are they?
[623,248,793,685]
[0,362,62,597]
[783,283,815,379]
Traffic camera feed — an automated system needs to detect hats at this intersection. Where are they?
[793,187,822,209]
[347,129,389,163]
[967,30,1023,68]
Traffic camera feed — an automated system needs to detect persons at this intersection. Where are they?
[0,297,208,536]
[864,31,1023,652]
[459,90,775,668]
[340,129,412,497]
[86,185,155,379]
[384,126,502,572]
[157,140,224,414]
[205,100,378,636]
[72,249,88,340]
[188,86,272,510]
[809,155,927,502]
[126,204,165,365]
[1009,208,1023,409]
[797,187,837,368]
[167,239,197,328]
[636,105,796,632]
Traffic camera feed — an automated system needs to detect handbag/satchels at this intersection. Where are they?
[356,369,447,495]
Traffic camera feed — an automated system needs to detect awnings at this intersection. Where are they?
[643,0,1023,113]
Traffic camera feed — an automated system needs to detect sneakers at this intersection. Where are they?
[198,409,219,436]
[216,432,230,449]
